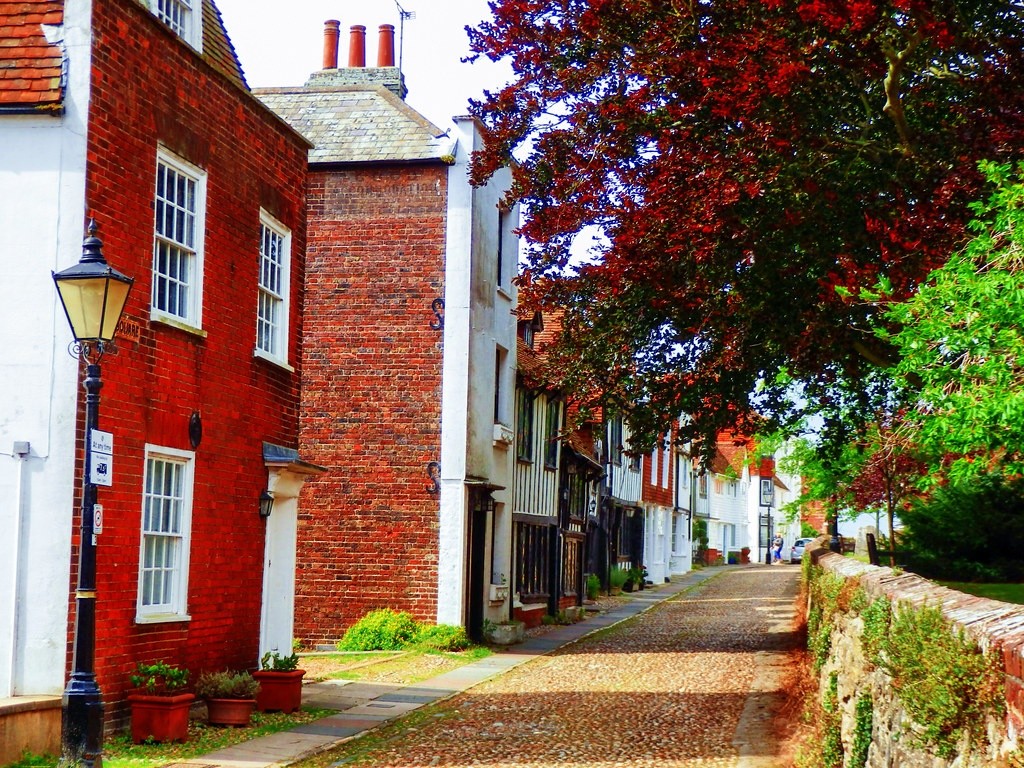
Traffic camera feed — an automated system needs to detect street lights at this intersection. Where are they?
[51,216,135,768]
[763,489,773,564]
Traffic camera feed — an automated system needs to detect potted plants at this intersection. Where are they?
[587,573,601,601]
[727,552,740,564]
[252,647,307,715]
[494,421,514,445]
[194,666,263,725]
[490,572,508,602]
[128,658,194,744]
[611,562,643,596]
[491,617,525,643]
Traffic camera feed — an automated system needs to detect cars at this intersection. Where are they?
[790,538,816,564]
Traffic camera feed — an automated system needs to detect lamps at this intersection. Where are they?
[625,507,636,517]
[260,488,274,516]
[589,497,596,513]
[562,488,569,500]
[482,491,496,511]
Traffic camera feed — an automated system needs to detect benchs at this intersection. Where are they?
[866,532,910,573]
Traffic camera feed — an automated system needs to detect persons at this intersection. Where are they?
[771,534,784,565]
